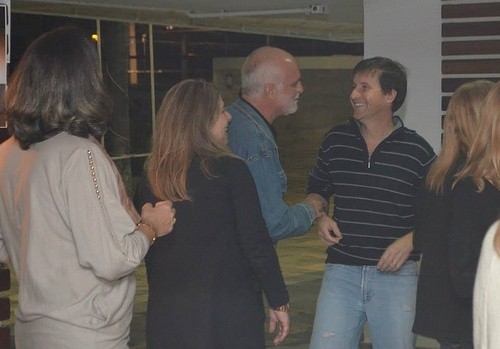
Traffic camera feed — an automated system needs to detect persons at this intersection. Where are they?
[410,78,500,349]
[222,45,328,239]
[134,78,291,349]
[308,55,438,349]
[0,27,176,349]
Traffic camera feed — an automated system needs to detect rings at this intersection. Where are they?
[390,260,397,267]
[173,218,178,224]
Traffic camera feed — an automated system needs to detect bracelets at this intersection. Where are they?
[135,219,159,244]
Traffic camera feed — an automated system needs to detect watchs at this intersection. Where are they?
[271,301,292,312]
